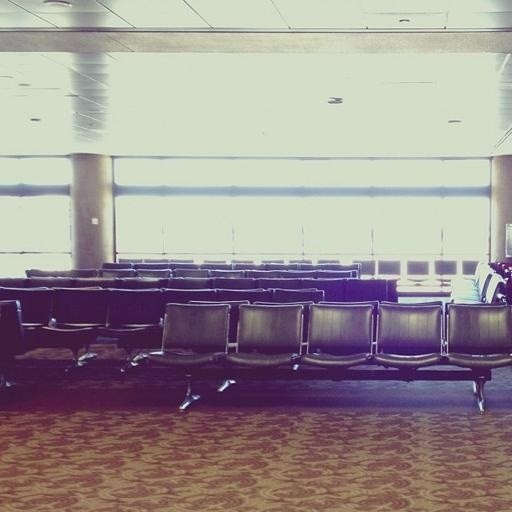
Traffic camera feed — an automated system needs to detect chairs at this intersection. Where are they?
[0,259,512,415]
